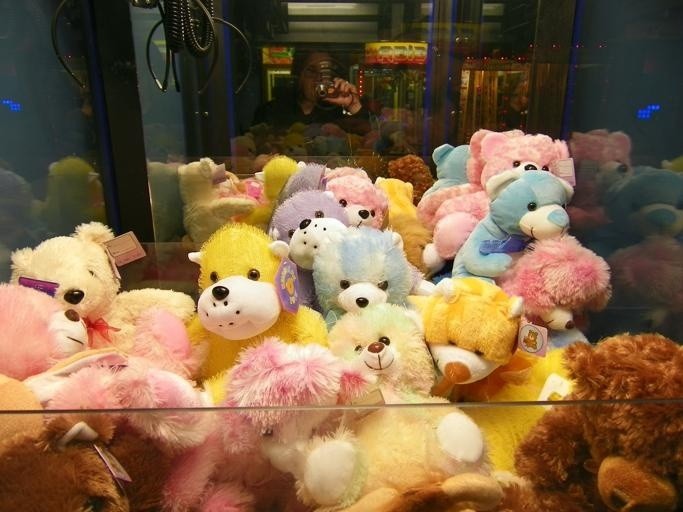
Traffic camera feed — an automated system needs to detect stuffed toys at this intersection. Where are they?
[0,128,682,512]
[1,126,683,512]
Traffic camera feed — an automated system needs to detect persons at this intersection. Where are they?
[249,42,383,155]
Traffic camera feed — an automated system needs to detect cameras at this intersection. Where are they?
[312,82,337,98]
